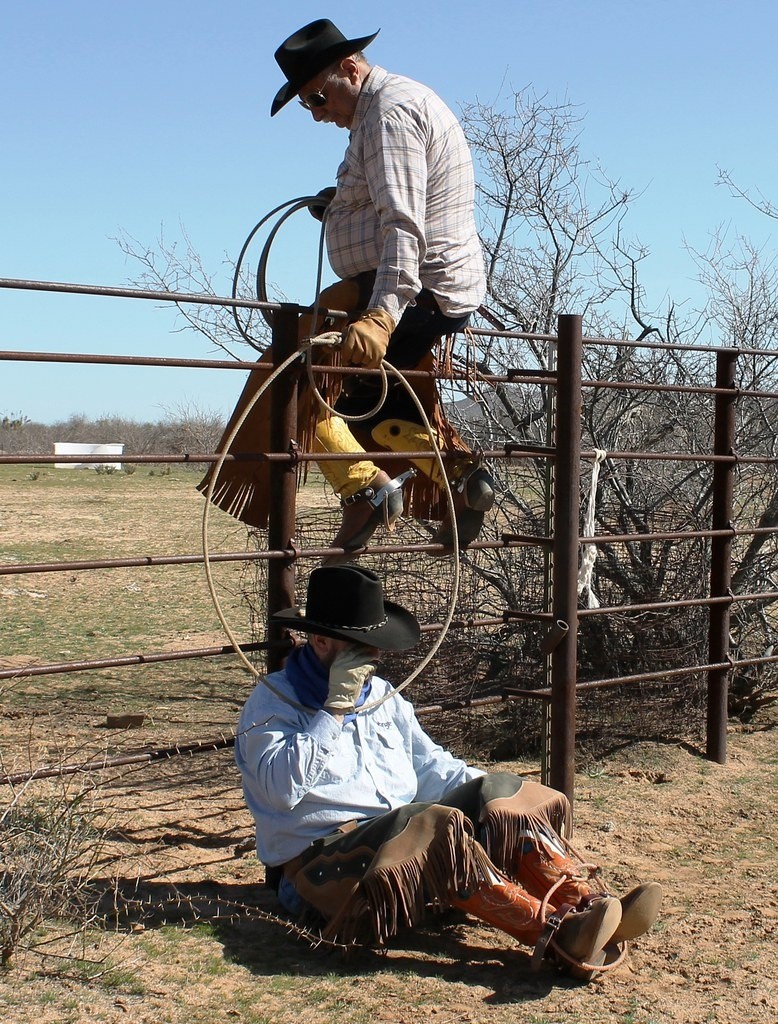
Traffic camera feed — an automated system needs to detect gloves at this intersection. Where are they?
[321,308,396,369]
[324,644,383,714]
[308,187,338,223]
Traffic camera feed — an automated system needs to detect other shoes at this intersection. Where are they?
[319,470,404,568]
[546,898,622,982]
[425,468,498,559]
[577,881,663,974]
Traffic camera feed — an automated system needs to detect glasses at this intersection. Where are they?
[298,66,336,110]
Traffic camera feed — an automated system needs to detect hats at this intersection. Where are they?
[270,18,381,117]
[273,565,420,650]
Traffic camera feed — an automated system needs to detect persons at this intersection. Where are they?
[235,565,663,983]
[270,17,496,567]
